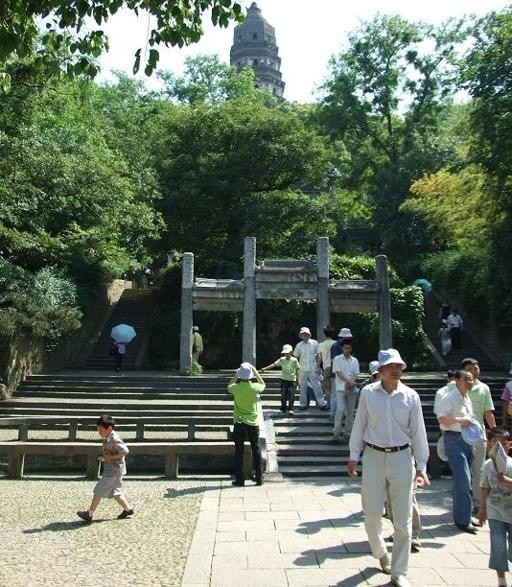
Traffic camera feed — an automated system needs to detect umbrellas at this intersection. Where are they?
[109,323,136,344]
[416,278,434,293]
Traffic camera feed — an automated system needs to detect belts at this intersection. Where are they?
[366,442,409,452]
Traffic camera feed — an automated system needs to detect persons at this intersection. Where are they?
[346,347,432,586]
[263,327,423,548]
[111,342,128,371]
[226,362,268,488]
[439,298,463,357]
[476,425,512,587]
[193,325,203,362]
[77,414,133,525]
[433,357,511,535]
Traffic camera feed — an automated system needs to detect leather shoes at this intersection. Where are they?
[459,523,479,531]
[232,480,244,487]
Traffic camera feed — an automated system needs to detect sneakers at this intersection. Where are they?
[412,538,422,547]
[380,553,391,573]
[117,510,133,519]
[77,511,91,522]
[299,407,307,410]
[391,576,409,587]
[320,405,329,410]
[288,410,294,415]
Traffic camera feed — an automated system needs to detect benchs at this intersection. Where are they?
[0,440,253,479]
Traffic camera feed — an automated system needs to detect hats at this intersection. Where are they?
[378,349,407,371]
[369,361,379,373]
[338,328,352,337]
[236,363,254,379]
[281,344,292,354]
[462,419,484,444]
[298,327,312,337]
[193,326,199,331]
